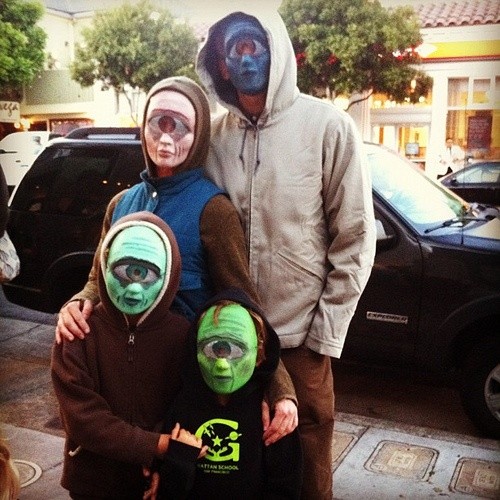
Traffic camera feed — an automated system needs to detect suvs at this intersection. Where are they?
[1,125,499,440]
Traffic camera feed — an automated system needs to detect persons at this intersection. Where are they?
[195,0,376,500]
[436,137,465,179]
[50,212,210,500]
[55,76,300,445]
[154,286,303,500]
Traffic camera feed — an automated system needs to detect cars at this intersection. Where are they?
[436,160,500,208]
